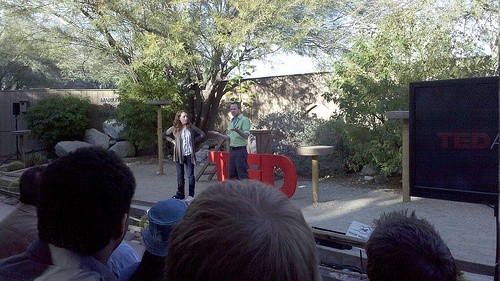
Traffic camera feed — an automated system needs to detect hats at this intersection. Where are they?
[140,197,190,257]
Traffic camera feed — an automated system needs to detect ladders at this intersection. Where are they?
[195,136,229,181]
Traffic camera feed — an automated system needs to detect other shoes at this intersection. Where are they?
[172,194,185,200]
[185,195,195,203]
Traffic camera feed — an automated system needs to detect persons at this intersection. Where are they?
[0,145,136,281]
[208,101,250,179]
[169,179,322,281]
[366,208,457,281]
[108,242,137,275]
[161,110,205,202]
[0,167,49,258]
[120,197,190,281]
